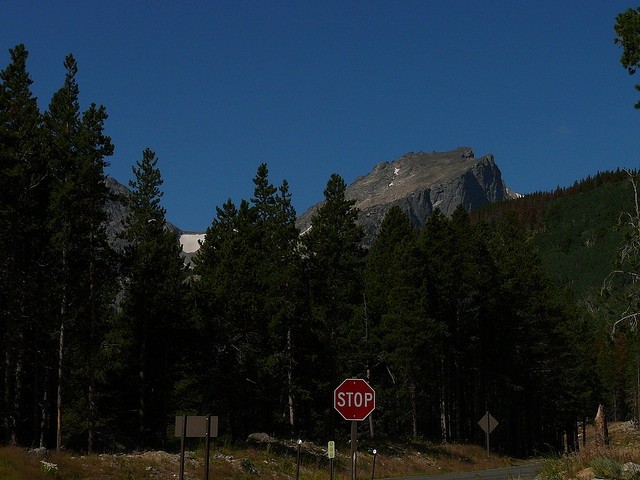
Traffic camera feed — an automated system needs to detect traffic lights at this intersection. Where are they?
[327,442,339,459]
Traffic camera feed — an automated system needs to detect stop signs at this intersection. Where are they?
[334,379,376,420]
